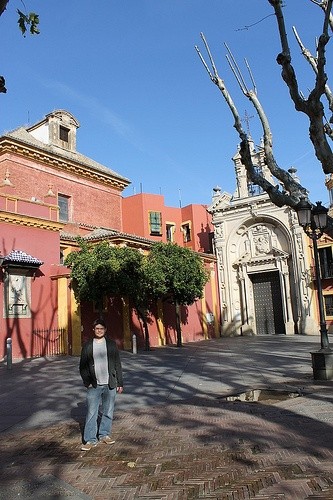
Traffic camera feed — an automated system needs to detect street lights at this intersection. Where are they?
[294,196,333,382]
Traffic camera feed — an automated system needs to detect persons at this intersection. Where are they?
[79,317,124,451]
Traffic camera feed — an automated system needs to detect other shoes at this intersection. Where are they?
[101,437,115,444]
[82,441,97,451]
